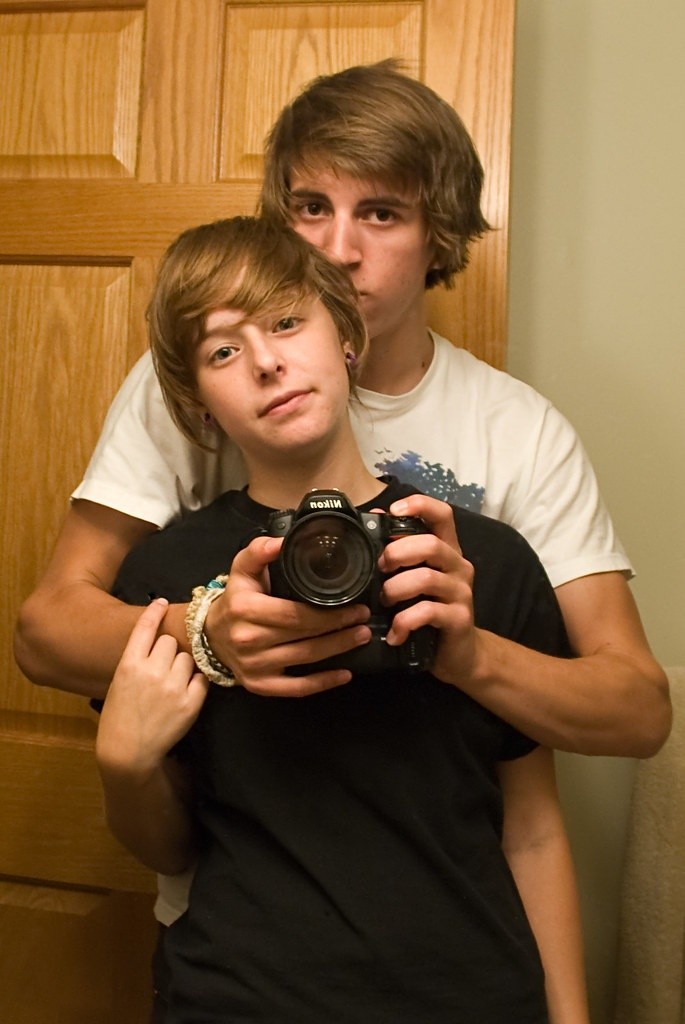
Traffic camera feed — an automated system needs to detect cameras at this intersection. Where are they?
[258,489,435,674]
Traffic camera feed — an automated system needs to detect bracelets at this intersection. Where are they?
[184,574,242,688]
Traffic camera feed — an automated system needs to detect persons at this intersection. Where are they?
[89,214,591,1023]
[9,54,673,1023]
